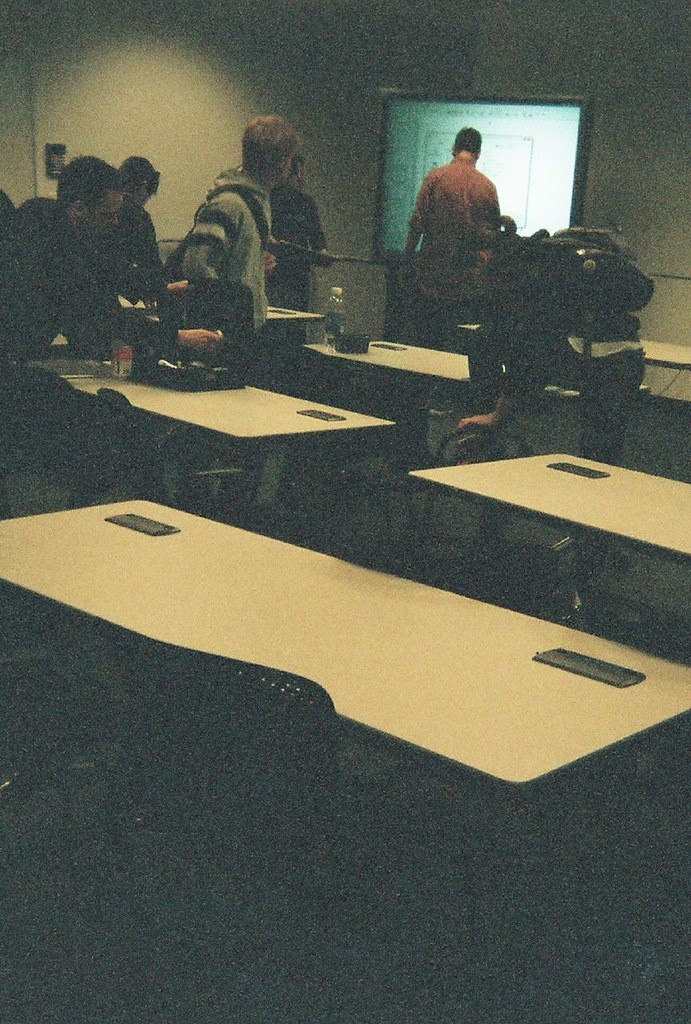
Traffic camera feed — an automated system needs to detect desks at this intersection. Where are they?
[264,305,325,343]
[407,451,691,592]
[0,498,691,788]
[303,341,507,450]
[457,324,691,372]
[24,356,397,527]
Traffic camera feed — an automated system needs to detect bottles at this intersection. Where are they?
[324,286,348,347]
[110,337,133,381]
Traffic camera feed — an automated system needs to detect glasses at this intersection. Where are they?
[291,168,309,176]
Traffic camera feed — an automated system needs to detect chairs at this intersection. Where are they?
[82,387,246,527]
[21,370,86,510]
[415,425,556,612]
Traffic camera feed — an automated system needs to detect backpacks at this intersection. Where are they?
[535,236,654,315]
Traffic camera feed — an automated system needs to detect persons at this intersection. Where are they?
[456,217,646,465]
[407,127,500,351]
[0,113,333,393]
